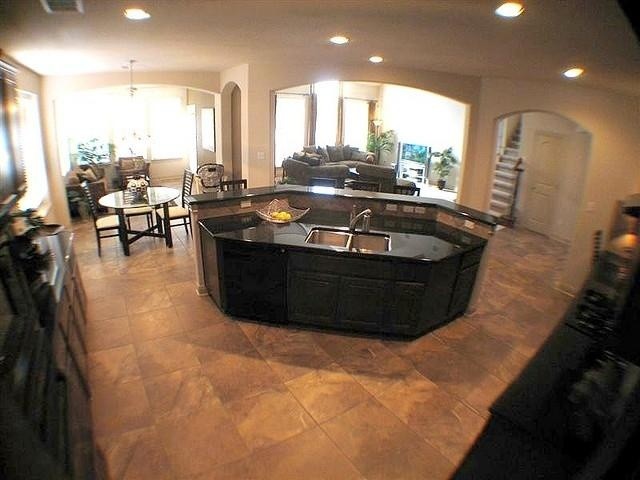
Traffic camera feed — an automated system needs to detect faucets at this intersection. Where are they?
[349,209,372,230]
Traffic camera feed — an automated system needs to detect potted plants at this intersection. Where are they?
[427,146,460,190]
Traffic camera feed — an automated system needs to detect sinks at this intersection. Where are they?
[349,235,390,252]
[305,228,349,249]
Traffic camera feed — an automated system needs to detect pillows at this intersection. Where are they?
[69,165,99,184]
[118,158,145,170]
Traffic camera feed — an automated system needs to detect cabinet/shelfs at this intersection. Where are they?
[445,246,484,324]
[1,231,94,479]
[285,245,426,341]
[449,250,639,478]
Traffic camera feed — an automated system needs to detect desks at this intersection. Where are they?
[98,187,181,256]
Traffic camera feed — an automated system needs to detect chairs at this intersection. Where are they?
[195,163,247,193]
[114,155,151,189]
[121,168,195,237]
[356,163,416,195]
[349,181,421,196]
[79,180,127,257]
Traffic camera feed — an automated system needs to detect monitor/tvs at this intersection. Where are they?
[402,143,427,163]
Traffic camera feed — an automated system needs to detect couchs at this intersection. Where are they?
[287,144,376,186]
[64,163,111,219]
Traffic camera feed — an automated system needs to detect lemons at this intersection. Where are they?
[272,211,291,220]
